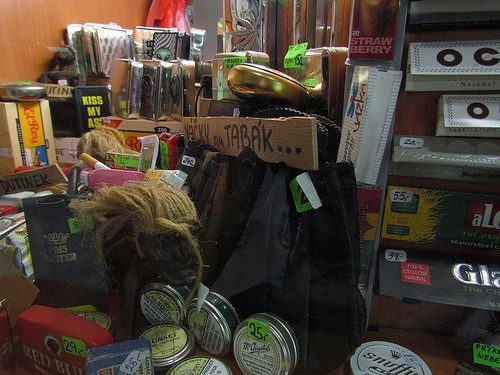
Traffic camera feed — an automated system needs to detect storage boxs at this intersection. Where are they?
[1,99,56,177]
